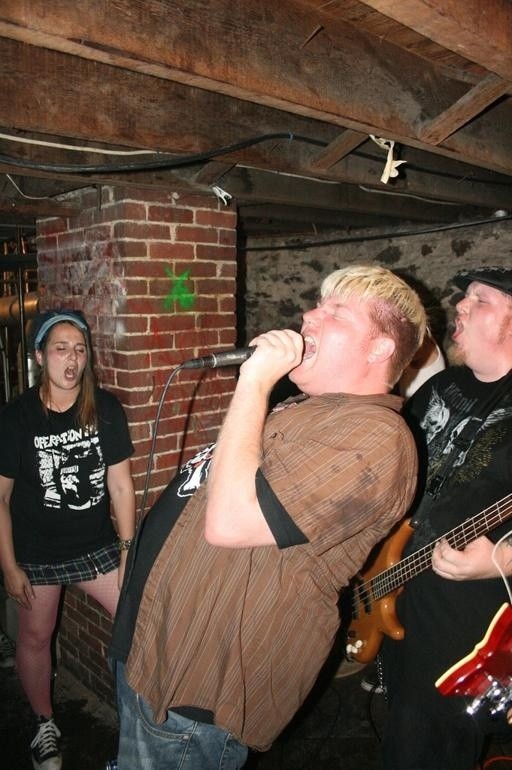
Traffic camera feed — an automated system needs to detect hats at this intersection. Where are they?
[26,308,87,354]
[452,269,512,293]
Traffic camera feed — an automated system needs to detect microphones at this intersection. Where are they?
[181,345,257,370]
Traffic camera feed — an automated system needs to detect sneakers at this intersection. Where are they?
[26,713,62,770]
[0,634,14,668]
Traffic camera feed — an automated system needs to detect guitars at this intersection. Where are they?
[332,494,512,680]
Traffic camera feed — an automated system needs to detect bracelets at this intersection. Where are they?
[117,540,133,550]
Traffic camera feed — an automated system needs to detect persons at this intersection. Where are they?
[382,270,512,770]
[0,310,136,770]
[108,265,420,770]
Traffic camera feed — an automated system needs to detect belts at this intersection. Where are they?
[166,704,216,724]
